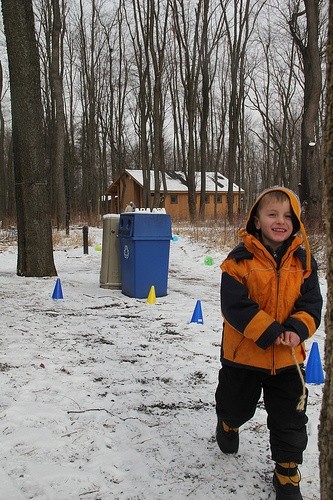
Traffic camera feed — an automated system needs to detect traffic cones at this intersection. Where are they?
[51,278,63,300]
[304,342,325,384]
[189,299,204,325]
[146,284,157,305]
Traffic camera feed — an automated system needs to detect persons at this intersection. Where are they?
[214,188,323,500]
[124,201,136,212]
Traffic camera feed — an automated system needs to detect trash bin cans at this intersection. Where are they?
[118,208,173,299]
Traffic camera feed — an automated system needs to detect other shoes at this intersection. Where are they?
[273,462,303,500]
[216,417,240,456]
